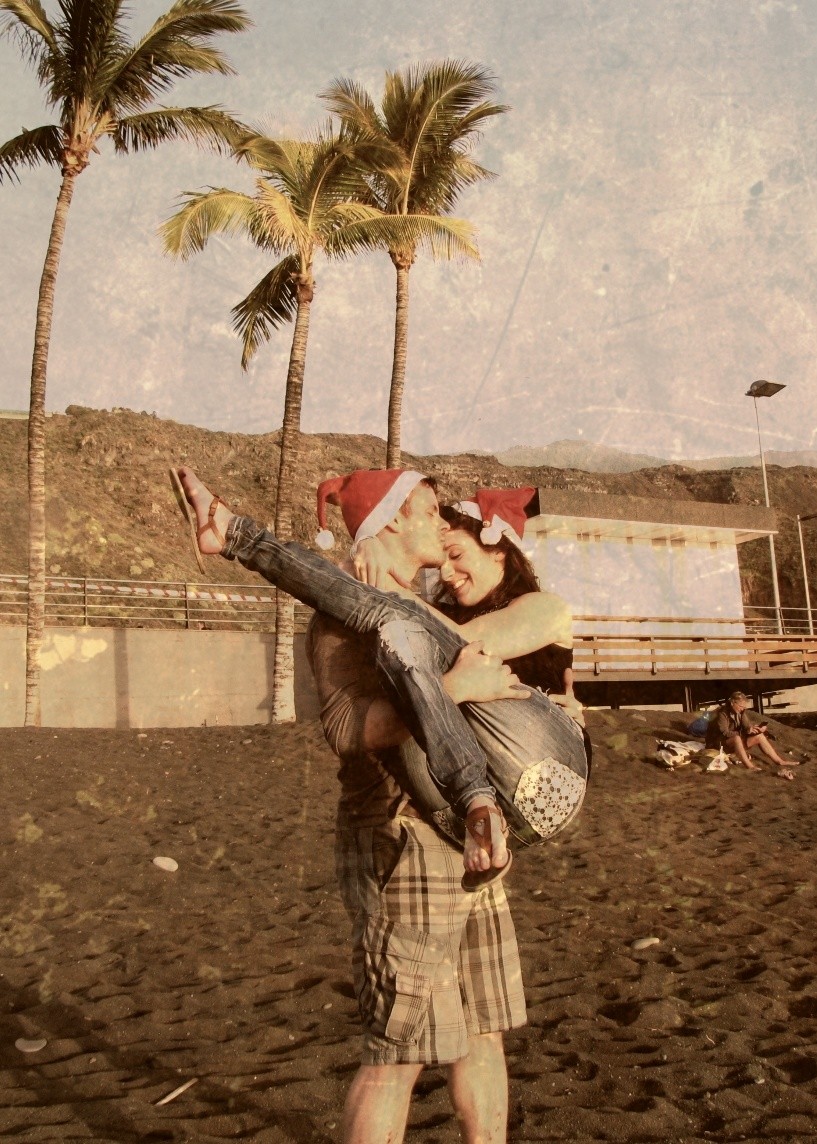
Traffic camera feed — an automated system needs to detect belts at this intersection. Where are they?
[350,799,425,819]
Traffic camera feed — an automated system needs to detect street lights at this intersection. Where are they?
[797,511,817,636]
[746,379,788,633]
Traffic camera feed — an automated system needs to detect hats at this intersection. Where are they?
[316,469,427,551]
[449,488,536,548]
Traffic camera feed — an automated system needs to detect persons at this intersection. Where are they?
[166,459,596,895]
[704,690,801,770]
[302,465,530,1144]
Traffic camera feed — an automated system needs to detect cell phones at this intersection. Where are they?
[759,722,768,727]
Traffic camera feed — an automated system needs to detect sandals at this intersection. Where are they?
[168,466,233,575]
[460,805,513,893]
